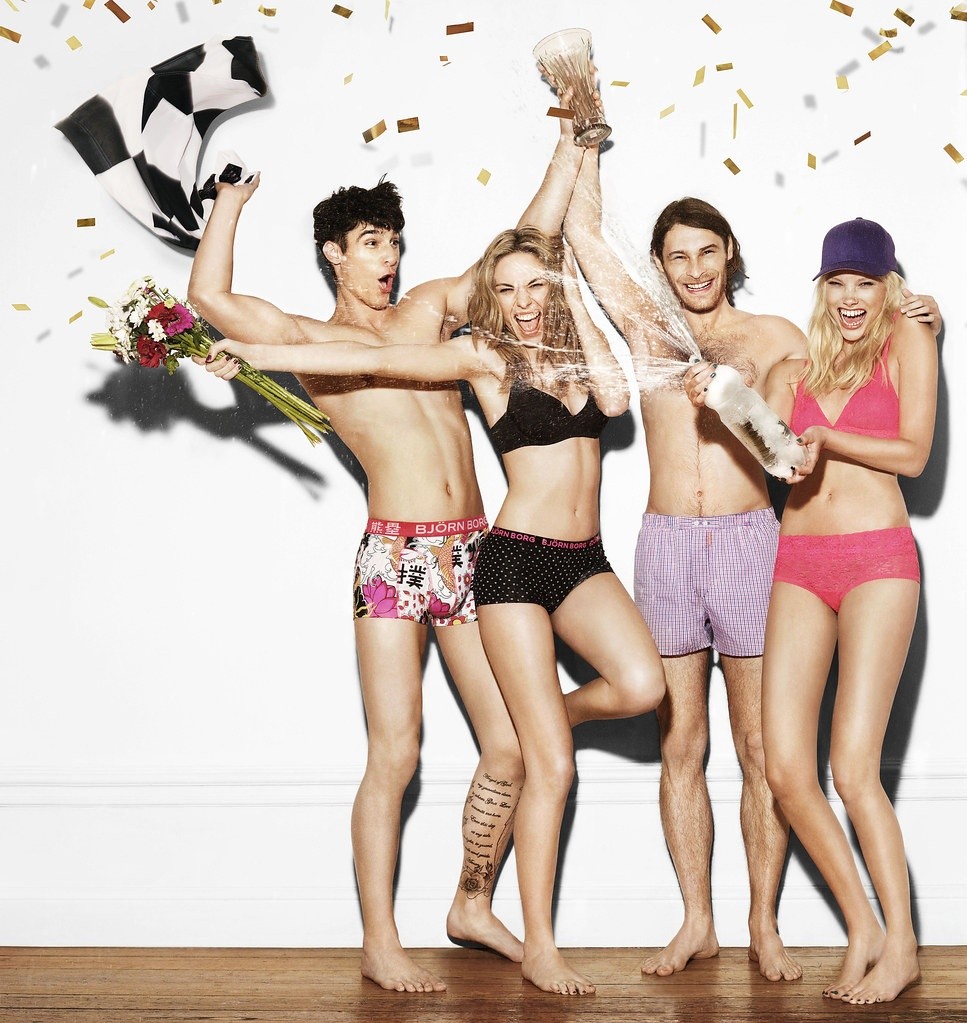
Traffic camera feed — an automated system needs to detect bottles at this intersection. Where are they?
[688,353,812,482]
[532,26,612,147]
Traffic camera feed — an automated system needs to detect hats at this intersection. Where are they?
[812,216,899,282]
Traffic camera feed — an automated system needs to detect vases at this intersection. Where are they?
[531,27,614,149]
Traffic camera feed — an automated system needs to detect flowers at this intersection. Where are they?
[84,274,336,446]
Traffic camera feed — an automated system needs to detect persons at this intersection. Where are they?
[203,222,668,993]
[557,60,945,983]
[184,60,608,998]
[683,217,943,1008]
